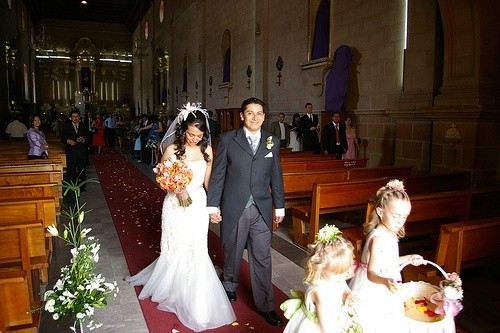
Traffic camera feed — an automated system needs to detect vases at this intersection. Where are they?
[118,115,124,121]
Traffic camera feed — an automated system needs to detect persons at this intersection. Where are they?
[272,104,359,161]
[278,180,427,333]
[206,98,286,327]
[0,109,217,192]
[123,101,237,332]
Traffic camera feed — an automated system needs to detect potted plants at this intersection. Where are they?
[70,108,80,117]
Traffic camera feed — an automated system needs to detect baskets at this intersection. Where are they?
[400,260,456,333]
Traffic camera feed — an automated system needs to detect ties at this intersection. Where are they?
[310,115,312,121]
[249,136,257,155]
[336,125,340,143]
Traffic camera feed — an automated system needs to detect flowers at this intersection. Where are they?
[146,137,159,149]
[42,169,120,323]
[98,106,107,115]
[266,135,275,149]
[432,272,463,316]
[115,108,124,116]
[153,159,194,207]
[318,224,339,247]
[386,180,408,192]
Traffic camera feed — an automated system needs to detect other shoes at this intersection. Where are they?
[261,310,283,326]
[226,290,237,302]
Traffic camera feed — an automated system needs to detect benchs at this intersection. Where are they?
[271,144,500,332]
[0,131,70,333]
